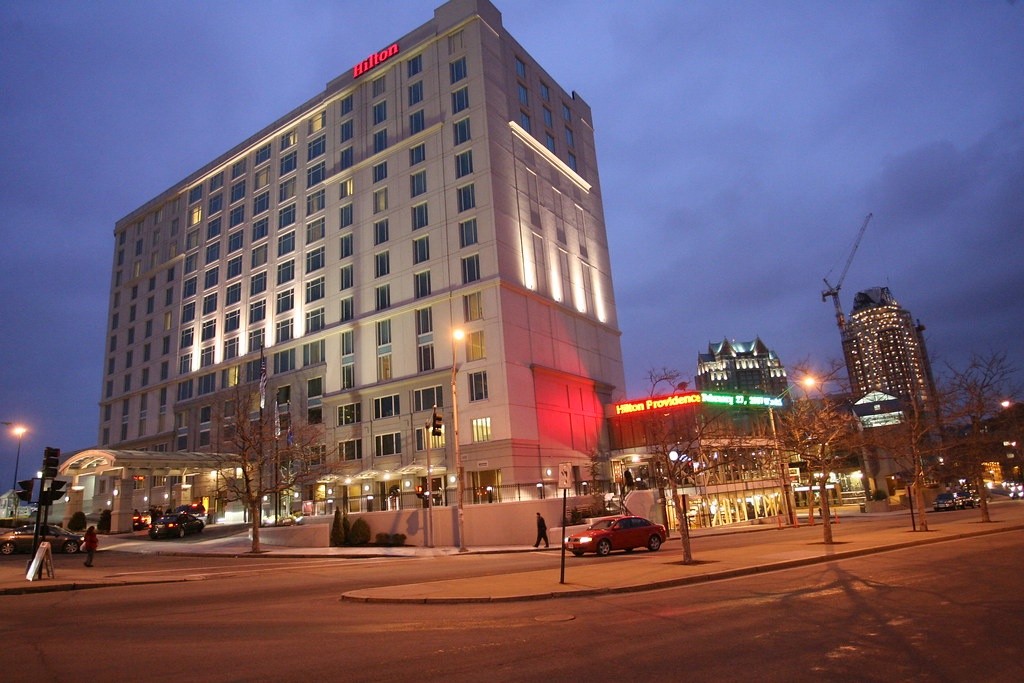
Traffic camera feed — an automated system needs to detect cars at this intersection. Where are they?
[0,523,86,556]
[148,512,204,539]
[565,514,667,556]
[932,491,979,511]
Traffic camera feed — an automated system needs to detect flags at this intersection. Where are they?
[259,356,268,409]
[287,420,293,445]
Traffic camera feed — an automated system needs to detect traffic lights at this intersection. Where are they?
[417,486,425,499]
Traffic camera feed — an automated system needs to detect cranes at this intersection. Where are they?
[821,213,875,337]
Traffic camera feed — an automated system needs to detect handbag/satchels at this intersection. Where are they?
[80,542,88,552]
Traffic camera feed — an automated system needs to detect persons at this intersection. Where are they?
[532,513,549,548]
[197,501,205,513]
[624,467,634,486]
[149,505,163,520]
[83,526,98,567]
[134,509,140,517]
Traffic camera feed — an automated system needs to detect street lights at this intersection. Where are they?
[12,428,24,489]
[451,331,465,551]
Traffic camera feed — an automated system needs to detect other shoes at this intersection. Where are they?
[533,544,538,547]
[544,545,549,547]
[84,563,93,567]
